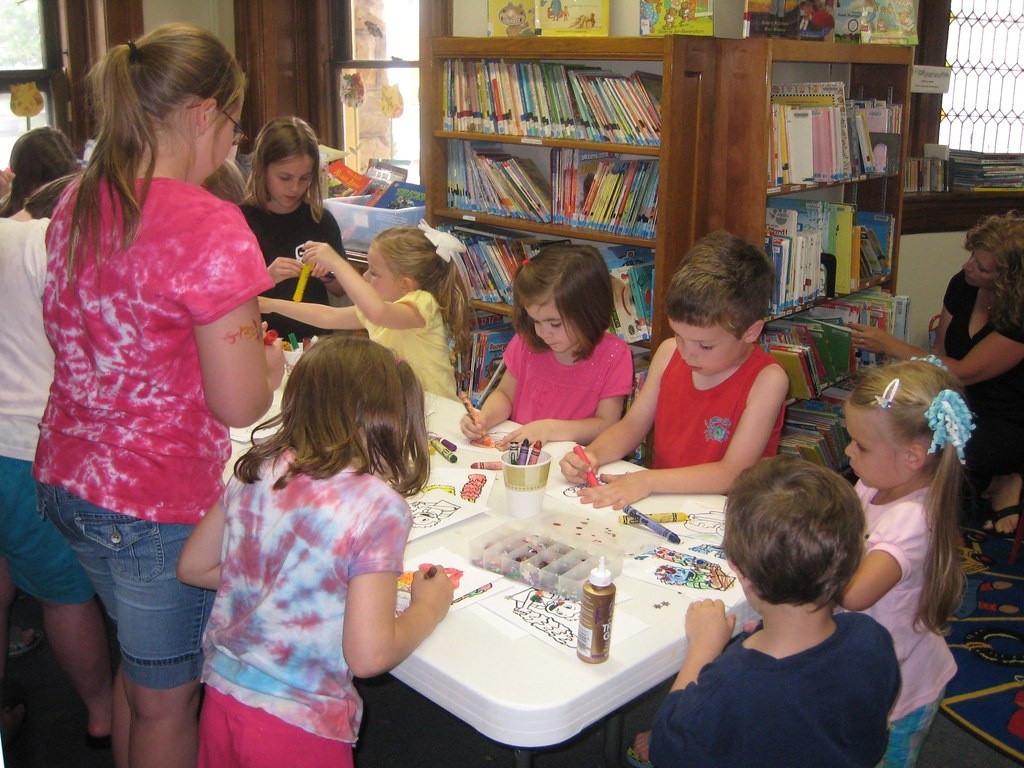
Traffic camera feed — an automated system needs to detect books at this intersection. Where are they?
[765,201,908,483]
[447,139,660,238]
[486,0,920,46]
[452,231,524,405]
[609,263,654,459]
[314,142,428,210]
[440,60,663,146]
[765,82,1024,194]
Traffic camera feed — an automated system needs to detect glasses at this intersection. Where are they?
[185,96,248,147]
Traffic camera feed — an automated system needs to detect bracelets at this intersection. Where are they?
[320,278,335,285]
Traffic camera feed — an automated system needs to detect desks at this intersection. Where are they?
[222,389,762,768]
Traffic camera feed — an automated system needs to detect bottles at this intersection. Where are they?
[577,557,616,664]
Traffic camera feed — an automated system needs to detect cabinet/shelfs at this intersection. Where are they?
[418,33,720,469]
[704,38,917,478]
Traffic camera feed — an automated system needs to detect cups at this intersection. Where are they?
[500,449,552,519]
[283,343,303,378]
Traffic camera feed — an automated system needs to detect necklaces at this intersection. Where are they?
[981,290,995,311]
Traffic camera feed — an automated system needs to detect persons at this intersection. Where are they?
[560,231,789,510]
[645,458,902,768]
[837,354,978,768]
[178,337,454,768]
[257,225,463,403]
[853,211,1024,534]
[198,116,347,341]
[460,242,634,452]
[0,23,284,768]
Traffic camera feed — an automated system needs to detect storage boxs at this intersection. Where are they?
[322,194,426,249]
[464,522,624,602]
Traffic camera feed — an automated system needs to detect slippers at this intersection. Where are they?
[981,502,1021,539]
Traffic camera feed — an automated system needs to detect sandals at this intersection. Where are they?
[8,625,44,657]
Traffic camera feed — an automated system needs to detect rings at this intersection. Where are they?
[858,338,864,344]
